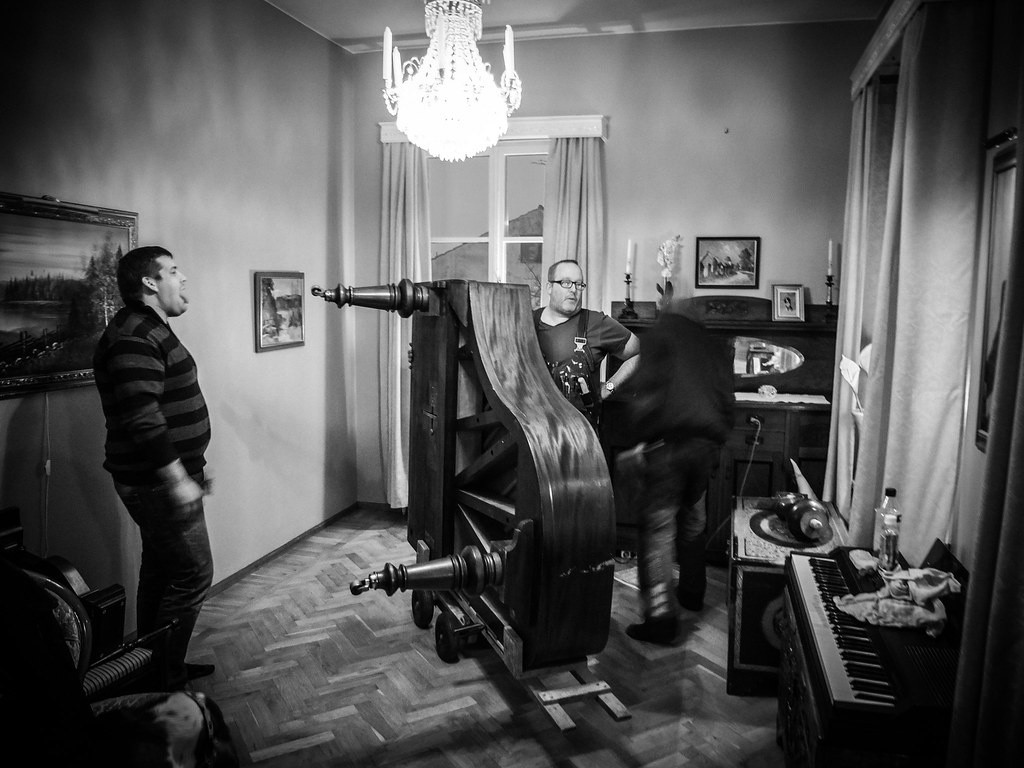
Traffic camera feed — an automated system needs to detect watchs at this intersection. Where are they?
[605,380,616,396]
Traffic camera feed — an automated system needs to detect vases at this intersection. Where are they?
[658,297,671,319]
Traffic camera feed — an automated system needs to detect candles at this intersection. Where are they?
[625,240,635,274]
[826,239,834,276]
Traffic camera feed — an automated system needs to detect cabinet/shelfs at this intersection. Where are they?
[704,393,847,573]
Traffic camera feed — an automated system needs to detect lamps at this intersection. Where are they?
[379,0,524,162]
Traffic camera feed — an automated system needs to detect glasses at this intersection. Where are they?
[550,279,588,290]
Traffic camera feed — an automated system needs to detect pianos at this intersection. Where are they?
[788,534,972,714]
[309,274,621,672]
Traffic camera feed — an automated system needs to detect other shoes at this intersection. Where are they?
[673,583,706,612]
[184,660,218,681]
[625,615,683,646]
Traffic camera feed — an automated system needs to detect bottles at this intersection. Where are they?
[878,514,898,570]
[873,487,901,551]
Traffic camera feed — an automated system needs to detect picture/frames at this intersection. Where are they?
[695,235,761,287]
[976,127,1021,454]
[254,273,308,351]
[0,194,138,403]
[772,284,806,321]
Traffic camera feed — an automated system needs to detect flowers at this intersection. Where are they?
[656,238,682,300]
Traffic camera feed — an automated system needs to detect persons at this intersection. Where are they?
[528,259,643,423]
[625,300,731,646]
[87,247,218,691]
